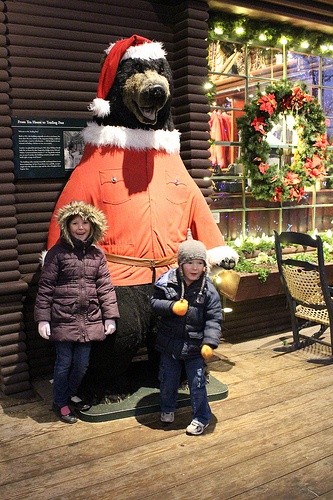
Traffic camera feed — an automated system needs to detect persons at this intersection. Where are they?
[147,229,224,436]
[33,200,120,424]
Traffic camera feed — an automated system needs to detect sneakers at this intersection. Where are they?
[187,420,209,435]
[52,402,76,422]
[69,395,90,410]
[160,412,174,422]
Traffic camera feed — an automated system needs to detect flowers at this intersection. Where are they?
[233,78,331,202]
[223,228,333,282]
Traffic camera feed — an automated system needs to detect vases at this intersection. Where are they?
[210,245,333,302]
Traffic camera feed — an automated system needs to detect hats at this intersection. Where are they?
[176,228,209,302]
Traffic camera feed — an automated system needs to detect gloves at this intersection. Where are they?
[201,345,213,359]
[104,319,116,335]
[172,298,189,316]
[38,321,51,340]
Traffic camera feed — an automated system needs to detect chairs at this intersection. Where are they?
[273,230,333,366]
